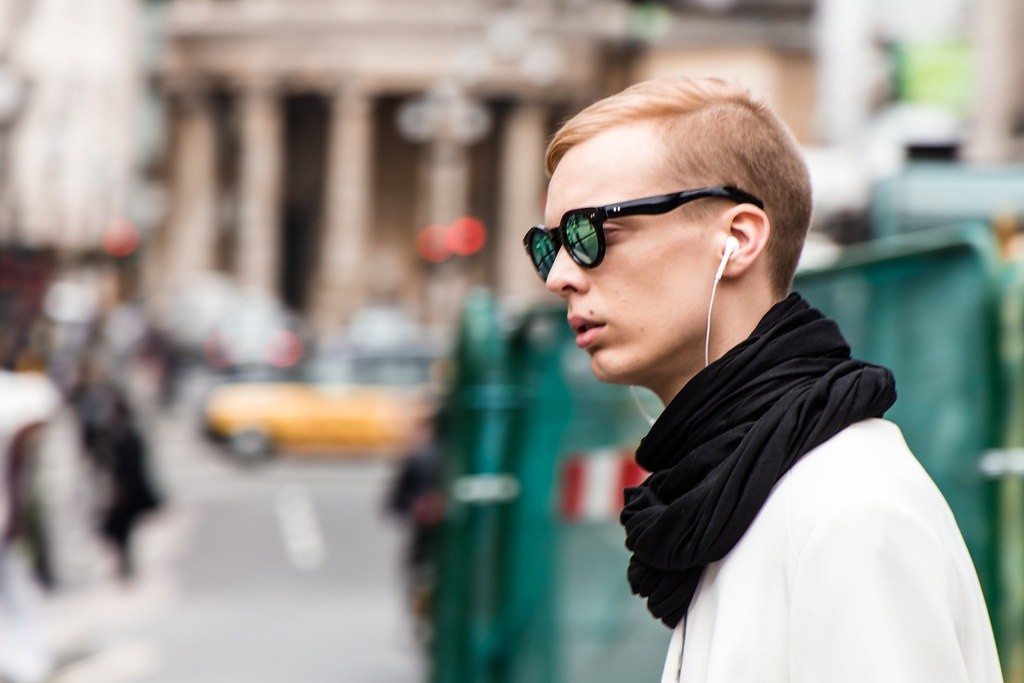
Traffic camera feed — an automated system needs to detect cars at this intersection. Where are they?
[201,355,433,467]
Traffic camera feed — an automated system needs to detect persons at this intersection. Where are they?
[523,74,1005,682]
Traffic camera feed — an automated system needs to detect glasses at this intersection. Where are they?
[522,184,764,285]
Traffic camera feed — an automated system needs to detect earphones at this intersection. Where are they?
[713,235,740,281]
